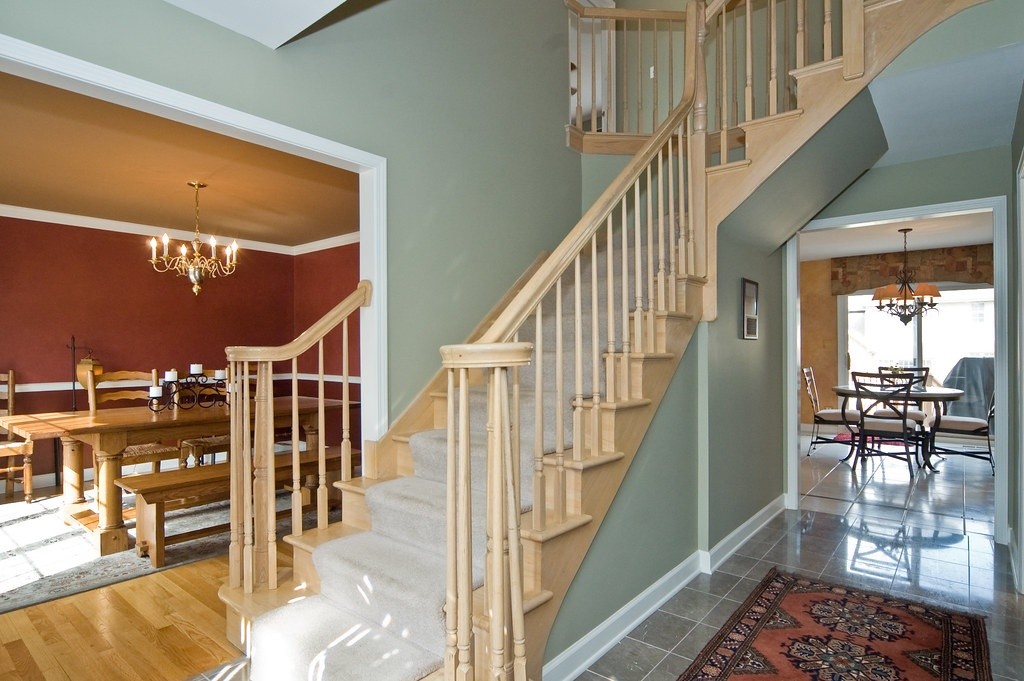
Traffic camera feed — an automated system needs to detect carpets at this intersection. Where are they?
[834,432,920,447]
[675,566,993,681]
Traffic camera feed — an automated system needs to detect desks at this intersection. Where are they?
[832,385,964,473]
[0,395,360,556]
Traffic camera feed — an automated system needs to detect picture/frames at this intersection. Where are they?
[741,277,759,340]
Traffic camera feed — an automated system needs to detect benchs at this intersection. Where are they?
[114,447,360,569]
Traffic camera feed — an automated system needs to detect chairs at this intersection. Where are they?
[227,365,258,399]
[0,369,32,505]
[850,371,918,479]
[87,369,182,512]
[802,367,872,458]
[168,369,231,468]
[921,392,995,476]
[870,366,930,454]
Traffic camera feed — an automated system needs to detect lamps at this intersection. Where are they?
[149,181,238,297]
[871,228,942,326]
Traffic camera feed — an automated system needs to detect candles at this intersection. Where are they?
[150,386,163,397]
[215,369,231,379]
[164,371,180,381]
[190,363,203,374]
